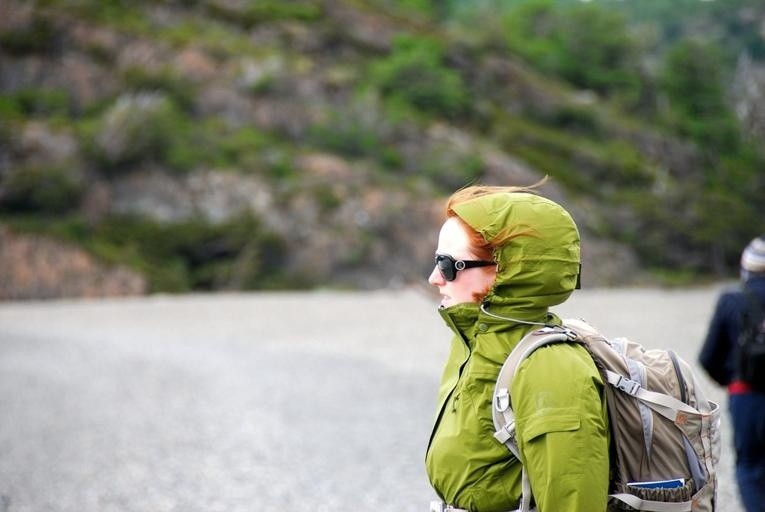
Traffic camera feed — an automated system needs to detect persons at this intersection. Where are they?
[425,173,614,512]
[698,237,765,512]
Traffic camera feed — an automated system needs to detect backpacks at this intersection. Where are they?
[493,316,723,511]
[736,287,765,393]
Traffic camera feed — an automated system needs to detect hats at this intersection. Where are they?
[741,237,765,273]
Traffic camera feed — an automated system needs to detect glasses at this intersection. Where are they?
[435,250,497,281]
